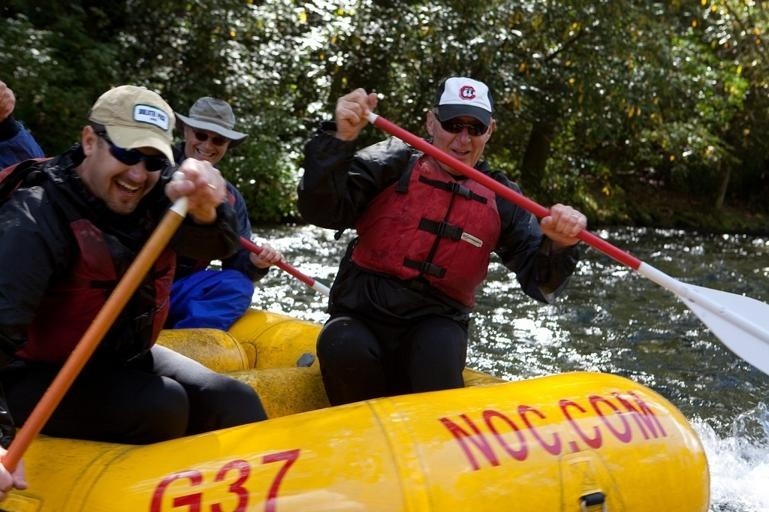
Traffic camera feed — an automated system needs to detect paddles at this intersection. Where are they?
[364,111,769,375]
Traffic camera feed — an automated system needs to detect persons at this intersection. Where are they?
[0,79,46,169]
[298,75,588,409]
[168,92,281,332]
[0,84,270,502]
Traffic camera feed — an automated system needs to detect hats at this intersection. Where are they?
[434,77,493,127]
[89,85,176,168]
[175,97,249,148]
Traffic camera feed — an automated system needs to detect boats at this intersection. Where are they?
[0,308,711,512]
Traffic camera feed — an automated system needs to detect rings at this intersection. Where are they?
[206,183,218,191]
[571,215,578,219]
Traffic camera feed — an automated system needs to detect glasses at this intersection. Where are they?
[97,131,166,171]
[435,114,488,136]
[190,126,225,146]
